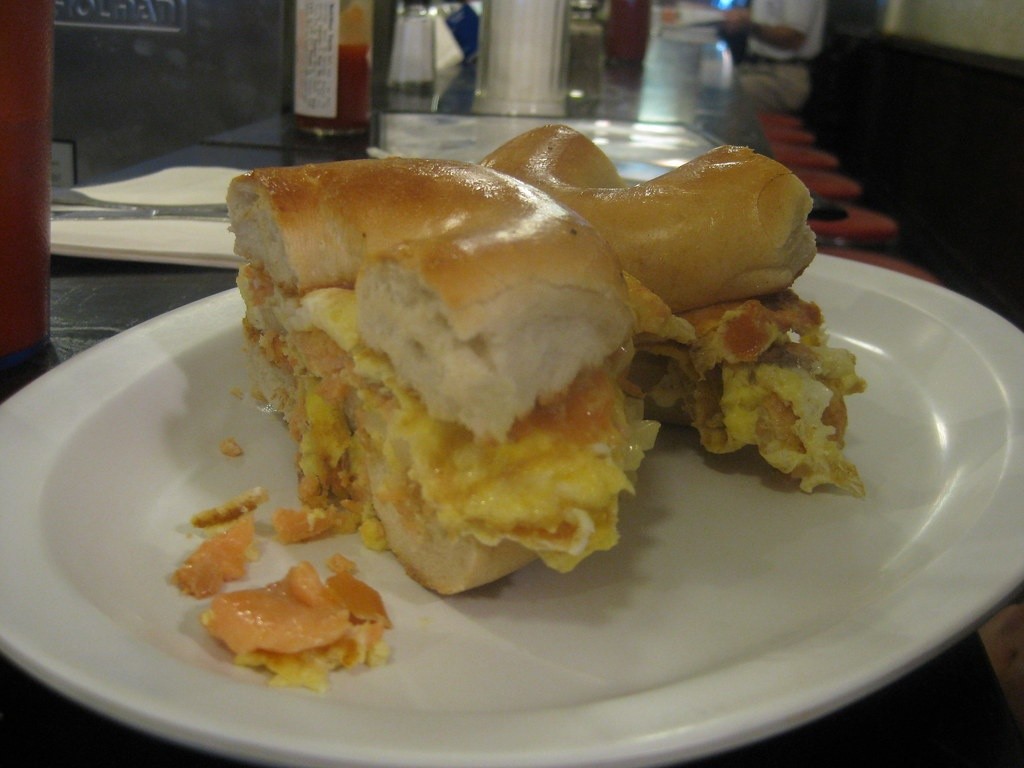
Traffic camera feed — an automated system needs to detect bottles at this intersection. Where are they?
[565,0,603,117]
[1,1,50,371]
[282,1,376,143]
[386,0,440,113]
[471,0,570,117]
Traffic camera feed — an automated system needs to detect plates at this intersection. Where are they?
[0,252,1023,767]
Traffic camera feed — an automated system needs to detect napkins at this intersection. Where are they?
[50,168,250,270]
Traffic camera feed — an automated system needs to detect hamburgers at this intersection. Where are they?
[224,156,661,594]
[479,123,868,495]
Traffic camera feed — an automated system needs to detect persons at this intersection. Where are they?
[722,1,828,144]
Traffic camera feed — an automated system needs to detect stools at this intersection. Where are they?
[755,109,937,282]
